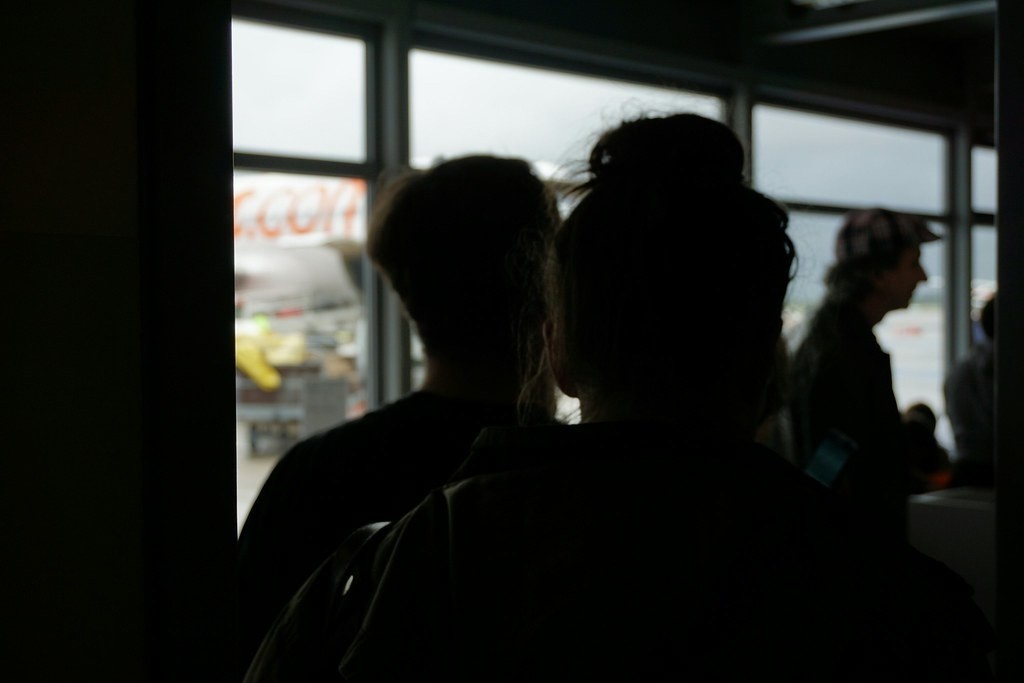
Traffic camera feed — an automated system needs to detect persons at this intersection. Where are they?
[944,294,996,486]
[890,404,958,484]
[236,155,566,598]
[786,212,971,600]
[253,113,997,683]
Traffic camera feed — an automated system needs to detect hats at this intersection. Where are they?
[837,209,938,263]
